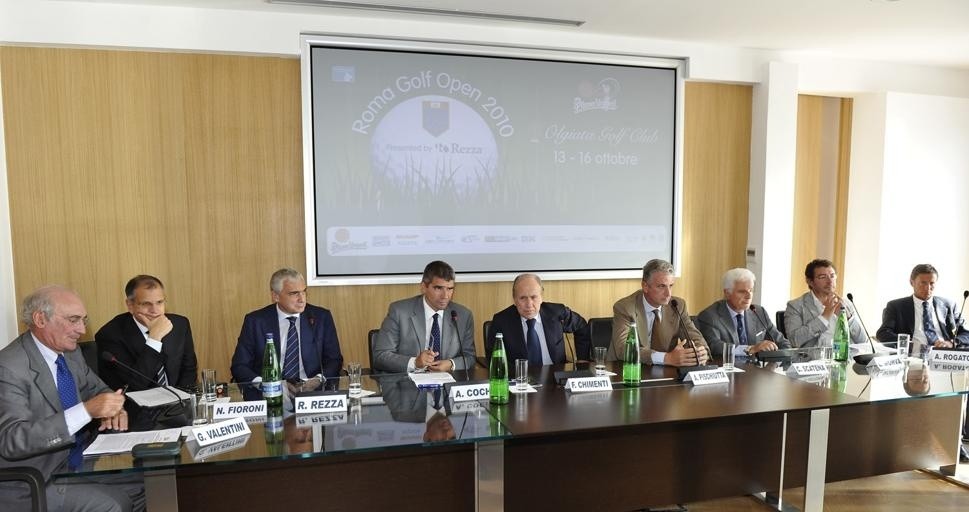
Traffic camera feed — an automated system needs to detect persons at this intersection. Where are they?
[377,369,474,442]
[230,267,343,401]
[693,268,792,358]
[371,261,477,374]
[486,274,591,382]
[883,342,932,397]
[605,259,714,367]
[242,377,339,454]
[95,275,197,395]
[876,264,969,350]
[0,285,146,512]
[783,259,866,348]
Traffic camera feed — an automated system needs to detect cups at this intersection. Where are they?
[190,396,208,425]
[595,347,607,374]
[201,368,217,400]
[723,343,734,371]
[516,359,528,390]
[897,334,909,356]
[347,361,361,395]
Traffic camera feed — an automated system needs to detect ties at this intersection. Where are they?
[281,316,300,385]
[647,309,666,351]
[921,301,938,346]
[735,312,748,345]
[429,313,440,362]
[157,362,165,387]
[526,319,543,368]
[55,352,85,468]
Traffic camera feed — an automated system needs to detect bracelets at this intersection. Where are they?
[746,348,753,357]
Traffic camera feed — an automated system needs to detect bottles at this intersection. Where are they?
[263,406,286,458]
[623,321,641,387]
[488,332,509,405]
[833,307,848,360]
[262,333,283,406]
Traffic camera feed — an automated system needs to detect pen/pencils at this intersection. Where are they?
[418,384,440,390]
[121,383,129,395]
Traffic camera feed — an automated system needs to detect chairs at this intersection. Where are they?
[484,321,493,362]
[369,330,377,360]
[776,311,787,339]
[691,316,701,330]
[79,342,99,375]
[588,318,613,350]
[0,467,47,512]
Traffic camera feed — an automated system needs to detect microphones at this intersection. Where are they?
[443,309,489,398]
[671,299,718,382]
[553,314,596,386]
[295,309,349,407]
[101,351,192,427]
[847,293,897,365]
[748,304,808,365]
[932,290,969,350]
